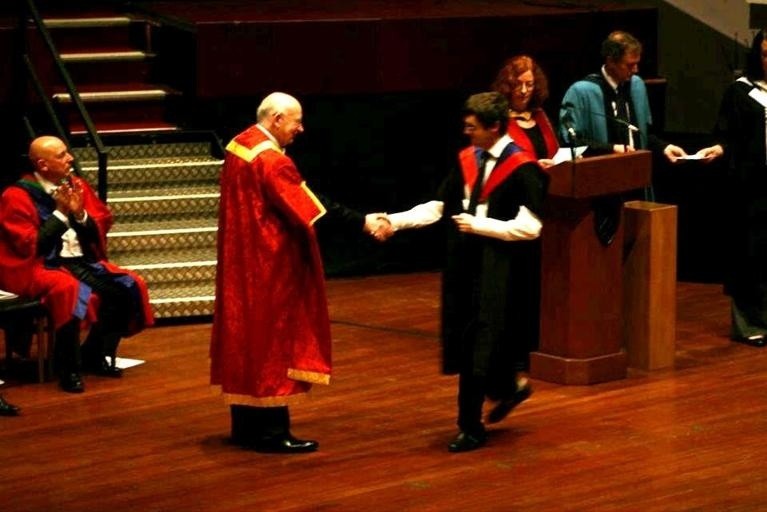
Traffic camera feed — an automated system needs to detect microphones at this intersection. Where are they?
[559,103,578,145]
[566,98,640,134]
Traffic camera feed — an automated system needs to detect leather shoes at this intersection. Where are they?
[94,365,121,377]
[60,368,82,391]
[254,430,319,453]
[0,398,18,415]
[448,428,485,452]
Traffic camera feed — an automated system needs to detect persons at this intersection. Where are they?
[0,135,155,393]
[210,91,391,454]
[492,55,584,170]
[370,92,552,454]
[693,27,767,346]
[556,33,687,204]
[2,398,22,418]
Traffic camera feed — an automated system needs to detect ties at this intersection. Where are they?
[468,155,488,215]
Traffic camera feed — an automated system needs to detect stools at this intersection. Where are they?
[6,296,51,382]
